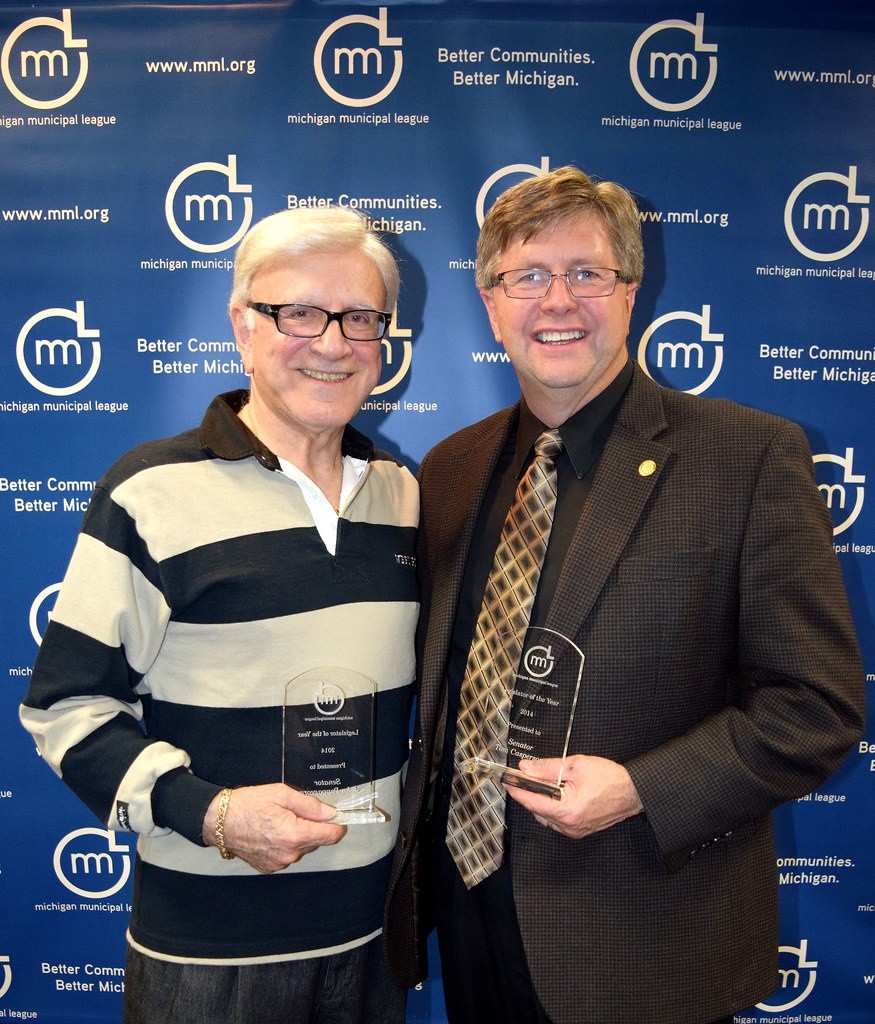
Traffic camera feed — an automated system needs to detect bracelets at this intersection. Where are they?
[218,788,236,863]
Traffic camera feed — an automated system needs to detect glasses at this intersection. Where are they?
[247,301,392,342]
[487,266,631,299]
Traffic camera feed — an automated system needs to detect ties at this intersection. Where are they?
[446,430,560,891]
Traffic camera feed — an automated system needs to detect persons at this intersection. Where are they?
[395,159,875,1024]
[16,204,441,1022]
